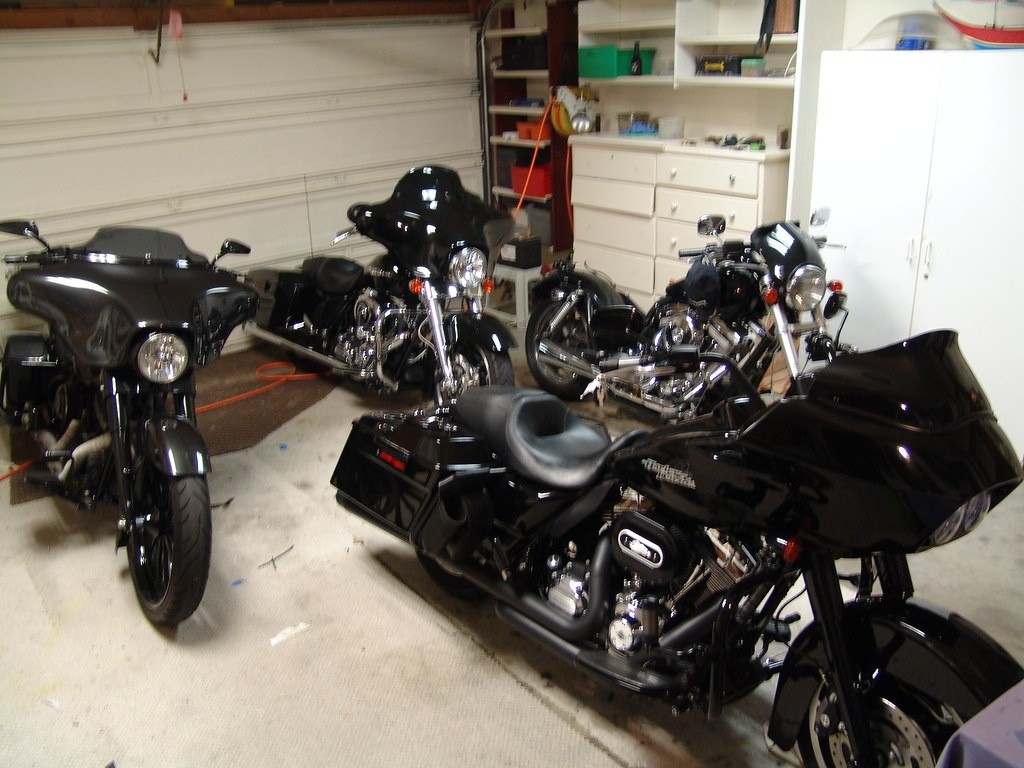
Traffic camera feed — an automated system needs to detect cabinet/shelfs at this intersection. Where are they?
[797,49,1024,467]
[484,27,555,254]
[577,0,848,223]
[568,131,792,315]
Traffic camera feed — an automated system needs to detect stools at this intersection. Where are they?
[484,263,548,330]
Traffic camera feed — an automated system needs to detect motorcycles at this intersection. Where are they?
[0,220,264,628]
[248,166,512,404]
[339,242,1023,768]
[524,207,860,437]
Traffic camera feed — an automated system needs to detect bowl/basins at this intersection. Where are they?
[741,59,765,77]
[617,113,648,132]
[658,119,684,138]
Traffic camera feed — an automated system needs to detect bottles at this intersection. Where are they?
[633,40,642,75]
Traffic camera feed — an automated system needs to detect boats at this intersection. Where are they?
[931,0,1024,49]
